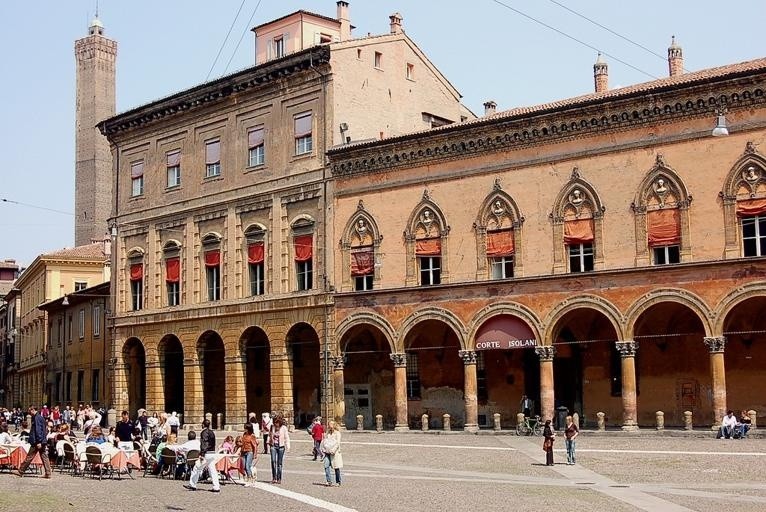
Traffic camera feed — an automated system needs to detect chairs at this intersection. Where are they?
[0,421,262,486]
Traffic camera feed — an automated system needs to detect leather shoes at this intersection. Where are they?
[12,471,22,476]
[39,475,51,477]
[183,485,196,490]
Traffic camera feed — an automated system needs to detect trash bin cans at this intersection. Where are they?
[557,406,569,430]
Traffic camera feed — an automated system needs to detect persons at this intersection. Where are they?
[657,179,667,193]
[573,190,582,203]
[720,409,737,439]
[320,421,343,487]
[1,403,201,479]
[307,416,326,461]
[746,167,759,181]
[493,201,504,215]
[520,394,535,436]
[735,409,752,439]
[423,210,432,223]
[564,415,580,465]
[223,423,257,482]
[250,413,273,454]
[357,219,366,232]
[183,419,220,492]
[543,419,556,466]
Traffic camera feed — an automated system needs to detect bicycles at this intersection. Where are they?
[515,416,546,437]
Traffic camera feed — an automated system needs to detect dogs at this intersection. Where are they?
[168,435,178,445]
[247,465,257,483]
